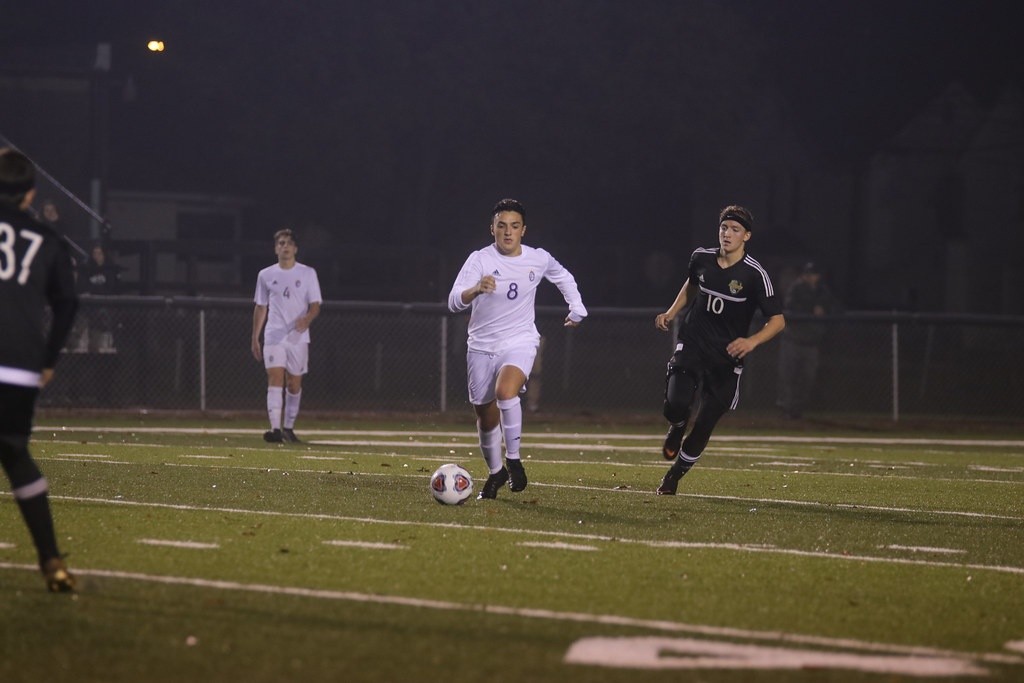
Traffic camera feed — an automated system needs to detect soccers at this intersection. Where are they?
[430,464,474,507]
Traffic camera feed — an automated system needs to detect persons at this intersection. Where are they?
[250,228,324,444]
[778,262,831,418]
[82,246,121,295]
[0,148,73,593]
[448,198,588,500]
[653,205,785,496]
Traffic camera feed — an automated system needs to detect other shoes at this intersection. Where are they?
[281,428,300,443]
[263,428,283,443]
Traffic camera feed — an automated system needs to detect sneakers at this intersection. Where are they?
[656,471,678,495]
[506,458,527,492]
[39,552,77,593]
[663,424,688,460]
[480,466,509,499]
